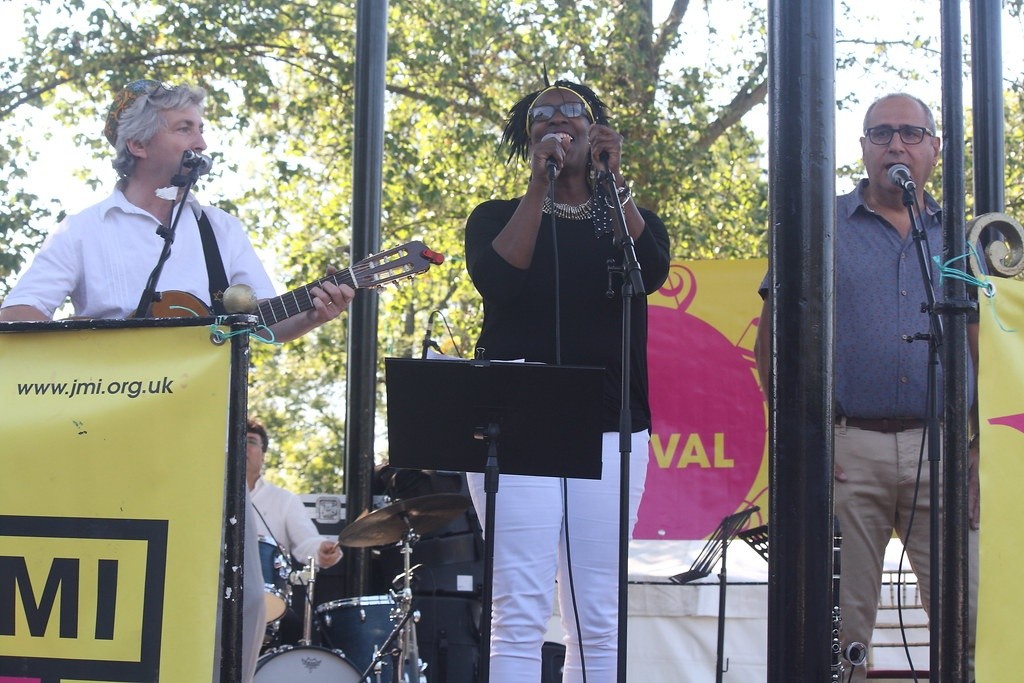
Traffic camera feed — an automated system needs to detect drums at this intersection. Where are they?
[256,532,293,628]
[248,642,370,683]
[312,593,426,683]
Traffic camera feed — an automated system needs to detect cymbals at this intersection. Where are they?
[338,492,472,549]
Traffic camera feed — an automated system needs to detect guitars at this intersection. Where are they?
[52,240,445,333]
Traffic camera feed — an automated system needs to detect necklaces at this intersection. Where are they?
[542,186,599,222]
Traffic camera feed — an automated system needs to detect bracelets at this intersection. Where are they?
[604,180,632,210]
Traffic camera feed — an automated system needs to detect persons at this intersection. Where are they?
[465,80,671,683]
[1,80,355,683]
[754,92,980,683]
[370,450,485,683]
[242,418,344,651]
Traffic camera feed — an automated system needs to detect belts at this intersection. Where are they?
[835,415,944,434]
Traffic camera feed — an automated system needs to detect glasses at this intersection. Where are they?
[150,80,177,99]
[528,101,592,125]
[865,127,933,146]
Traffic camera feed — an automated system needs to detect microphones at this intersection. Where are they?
[184,148,213,175]
[599,151,608,162]
[542,133,562,182]
[887,163,916,191]
[393,564,422,590]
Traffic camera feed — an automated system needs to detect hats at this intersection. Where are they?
[104,79,157,149]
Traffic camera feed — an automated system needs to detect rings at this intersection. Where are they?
[326,300,334,307]
